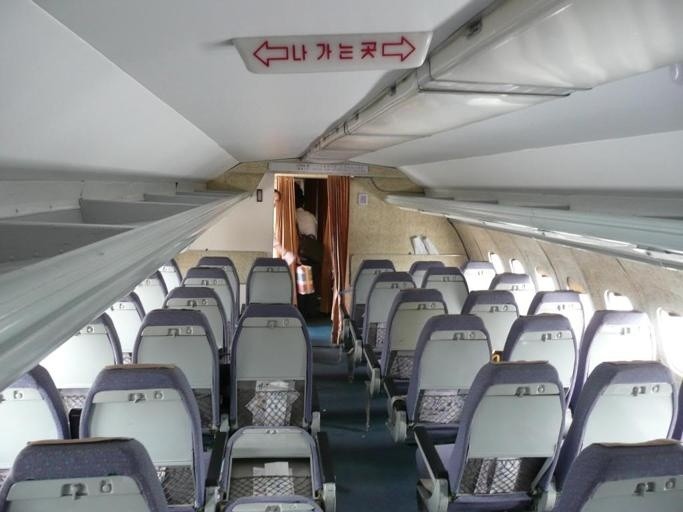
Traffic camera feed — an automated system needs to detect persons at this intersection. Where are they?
[295,193,319,320]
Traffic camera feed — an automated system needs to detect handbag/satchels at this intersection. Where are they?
[296,265,316,295]
[298,234,323,265]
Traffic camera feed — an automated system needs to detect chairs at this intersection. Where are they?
[73,363,212,507]
[550,439,681,508]
[559,362,677,476]
[220,495,318,507]
[3,367,70,470]
[413,363,561,508]
[7,439,168,507]
[219,425,324,502]
